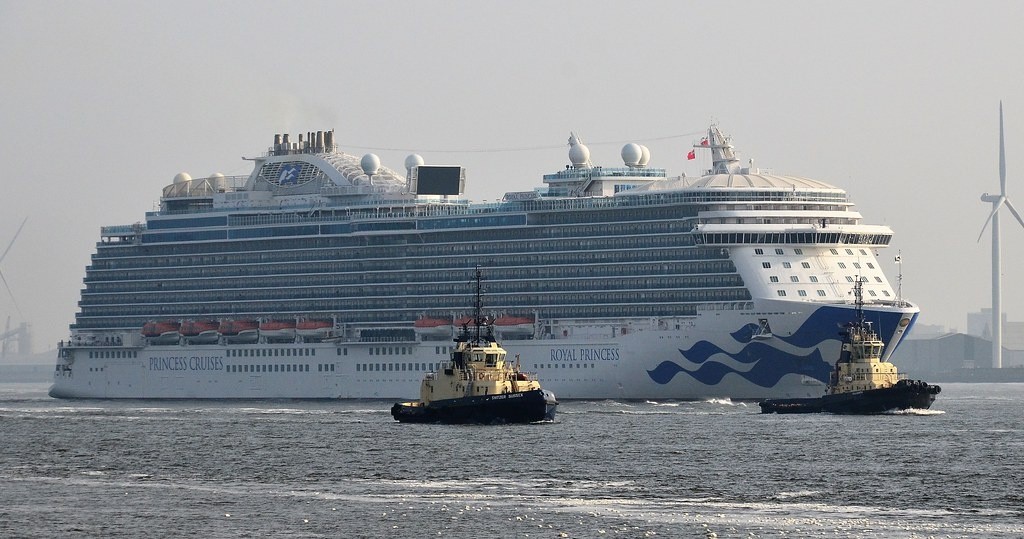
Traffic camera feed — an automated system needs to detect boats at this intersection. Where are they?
[759,275,941,414]
[391,270,559,425]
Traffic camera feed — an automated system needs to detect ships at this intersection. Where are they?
[48,117,920,399]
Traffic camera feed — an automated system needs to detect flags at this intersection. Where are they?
[701,138,709,146]
[687,149,695,160]
